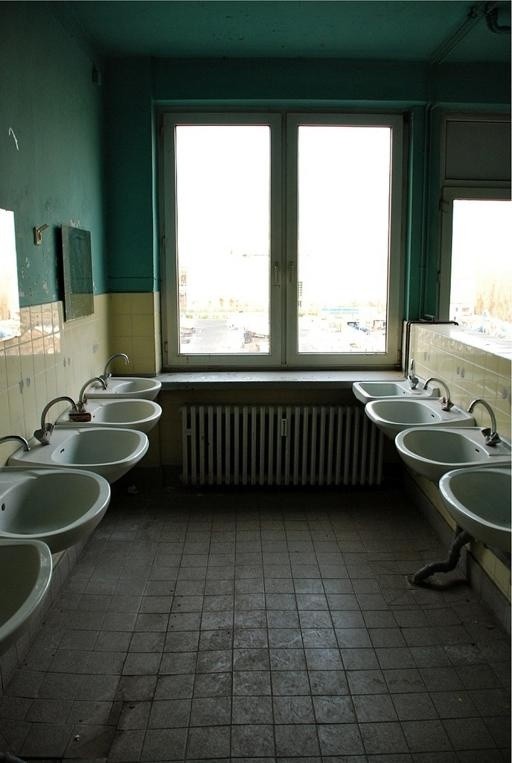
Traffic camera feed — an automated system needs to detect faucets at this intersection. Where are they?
[0,435,31,452]
[76,377,106,412]
[467,399,501,446]
[99,352,129,380]
[408,358,419,390]
[423,377,454,411]
[33,396,78,445]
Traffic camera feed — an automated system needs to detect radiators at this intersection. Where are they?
[182,405,384,486]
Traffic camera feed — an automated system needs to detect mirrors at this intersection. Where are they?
[0,209,22,341]
[61,224,94,322]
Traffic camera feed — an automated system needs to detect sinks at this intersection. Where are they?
[84,377,162,401]
[7,424,150,484]
[394,426,512,480]
[0,466,111,553]
[55,398,163,433]
[438,467,512,551]
[352,375,441,405]
[365,397,475,439]
[0,539,53,655]
